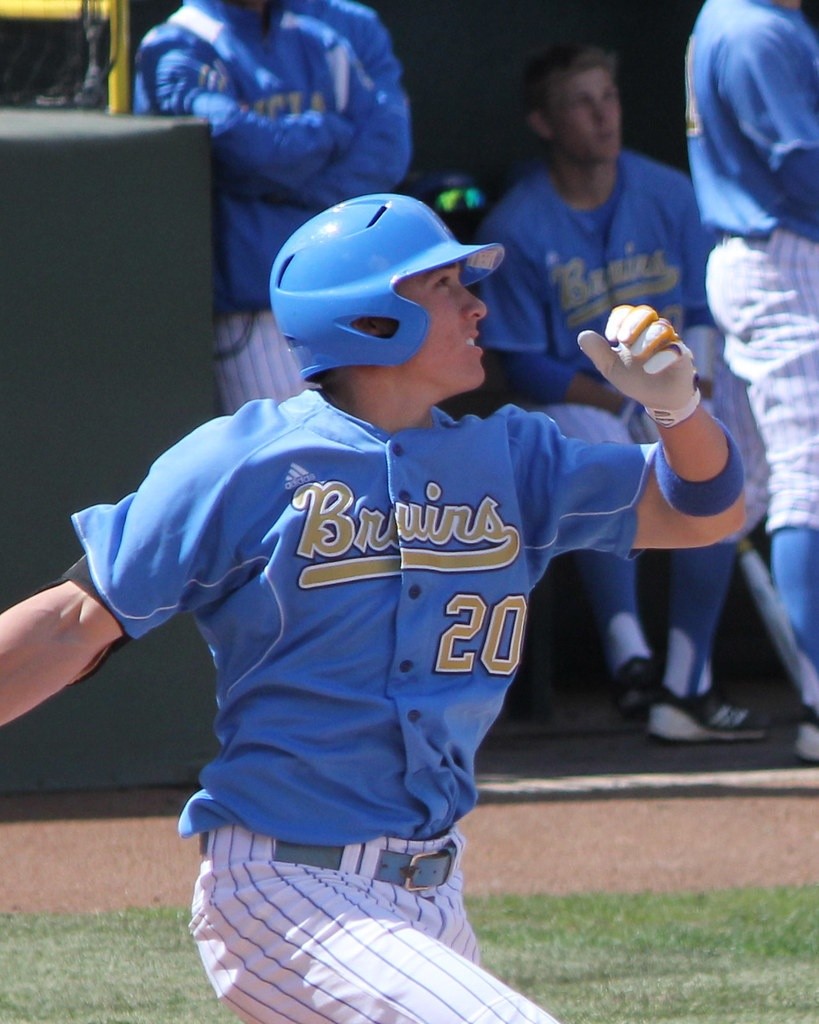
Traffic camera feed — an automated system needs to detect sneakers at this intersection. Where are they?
[645,684,766,738]
[618,652,657,706]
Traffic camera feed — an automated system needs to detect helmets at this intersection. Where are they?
[270,194,503,378]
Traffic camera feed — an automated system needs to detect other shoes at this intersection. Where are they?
[791,722,819,763]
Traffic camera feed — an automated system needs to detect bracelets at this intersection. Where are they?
[652,420,745,518]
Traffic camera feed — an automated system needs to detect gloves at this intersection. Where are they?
[578,304,700,426]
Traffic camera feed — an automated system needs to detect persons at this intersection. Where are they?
[682,0,819,761]
[475,42,770,743]
[0,192,747,1024]
[130,1,413,419]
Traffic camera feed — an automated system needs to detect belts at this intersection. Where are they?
[198,829,456,890]
